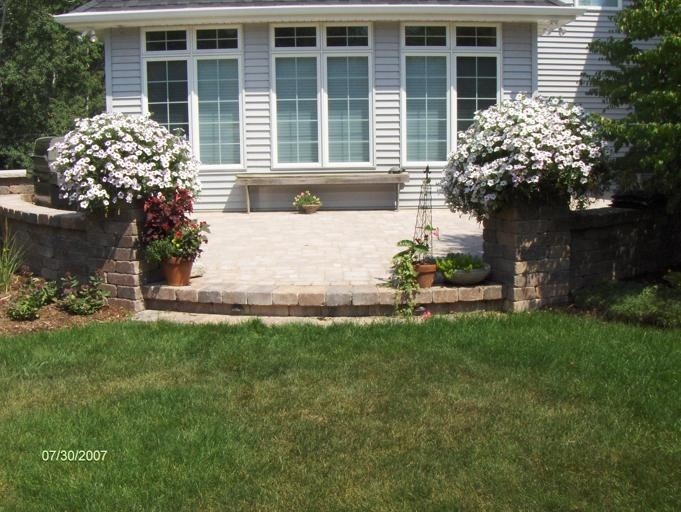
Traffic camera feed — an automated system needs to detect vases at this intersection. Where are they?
[411,261,437,289]
[162,257,194,286]
[301,204,320,213]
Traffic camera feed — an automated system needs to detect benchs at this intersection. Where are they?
[234,171,411,214]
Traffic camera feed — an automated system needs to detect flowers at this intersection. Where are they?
[436,86,614,223]
[46,108,204,220]
[373,223,440,325]
[292,191,322,208]
[138,187,212,264]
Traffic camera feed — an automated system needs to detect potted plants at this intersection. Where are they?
[435,251,491,285]
